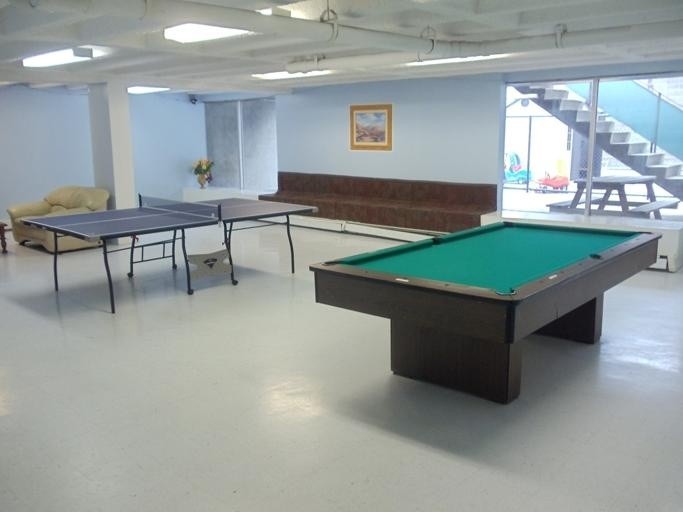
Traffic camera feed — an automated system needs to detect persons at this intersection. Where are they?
[509,152,520,173]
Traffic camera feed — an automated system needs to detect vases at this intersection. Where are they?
[197,173,207,189]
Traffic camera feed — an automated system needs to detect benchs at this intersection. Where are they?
[258,171,497,232]
[482,210,683,272]
[545,197,680,219]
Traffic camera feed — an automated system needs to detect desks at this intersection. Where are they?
[570,176,663,220]
[20,193,319,314]
[309,220,662,405]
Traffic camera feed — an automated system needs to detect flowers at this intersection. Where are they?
[191,159,216,184]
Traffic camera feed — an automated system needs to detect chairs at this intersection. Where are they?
[6,185,110,254]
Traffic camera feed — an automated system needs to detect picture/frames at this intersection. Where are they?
[349,104,393,151]
